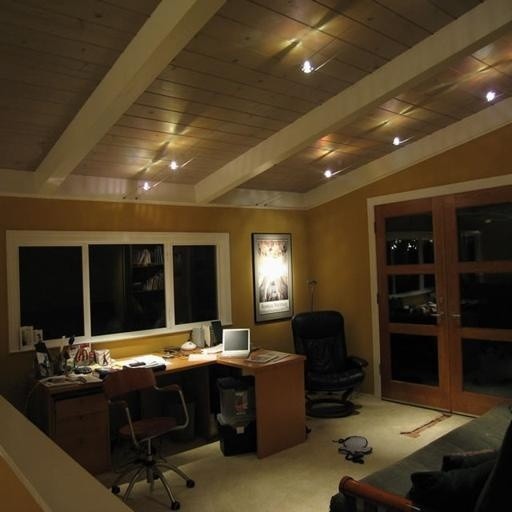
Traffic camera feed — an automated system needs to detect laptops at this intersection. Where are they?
[220,328,251,360]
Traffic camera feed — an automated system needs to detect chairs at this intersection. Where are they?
[292,311,368,417]
[101,367,195,510]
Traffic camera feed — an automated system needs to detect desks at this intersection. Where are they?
[28,343,308,477]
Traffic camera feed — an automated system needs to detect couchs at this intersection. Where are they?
[330,402,512,511]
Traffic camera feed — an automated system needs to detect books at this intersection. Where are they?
[246,350,290,363]
[193,319,223,348]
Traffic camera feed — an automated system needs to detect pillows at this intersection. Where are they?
[405,450,499,511]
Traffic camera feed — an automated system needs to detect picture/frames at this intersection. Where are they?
[252,233,293,323]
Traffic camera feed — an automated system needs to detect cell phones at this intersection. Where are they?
[129,361,146,367]
